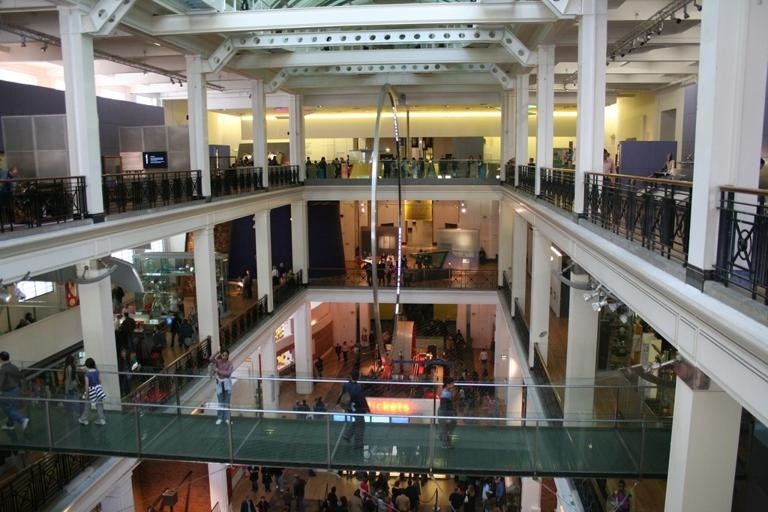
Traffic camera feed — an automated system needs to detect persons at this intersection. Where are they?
[660,153,676,174]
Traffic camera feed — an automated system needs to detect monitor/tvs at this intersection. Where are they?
[142,151,169,169]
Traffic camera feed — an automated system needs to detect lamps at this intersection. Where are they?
[561,0,702,90]
[580,277,634,328]
[0,271,33,305]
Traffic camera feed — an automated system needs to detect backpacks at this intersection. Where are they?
[340,383,358,411]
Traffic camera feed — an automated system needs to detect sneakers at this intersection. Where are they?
[78,417,89,426]
[225,418,233,425]
[94,418,106,425]
[216,418,224,425]
[1,424,16,430]
[22,416,30,433]
